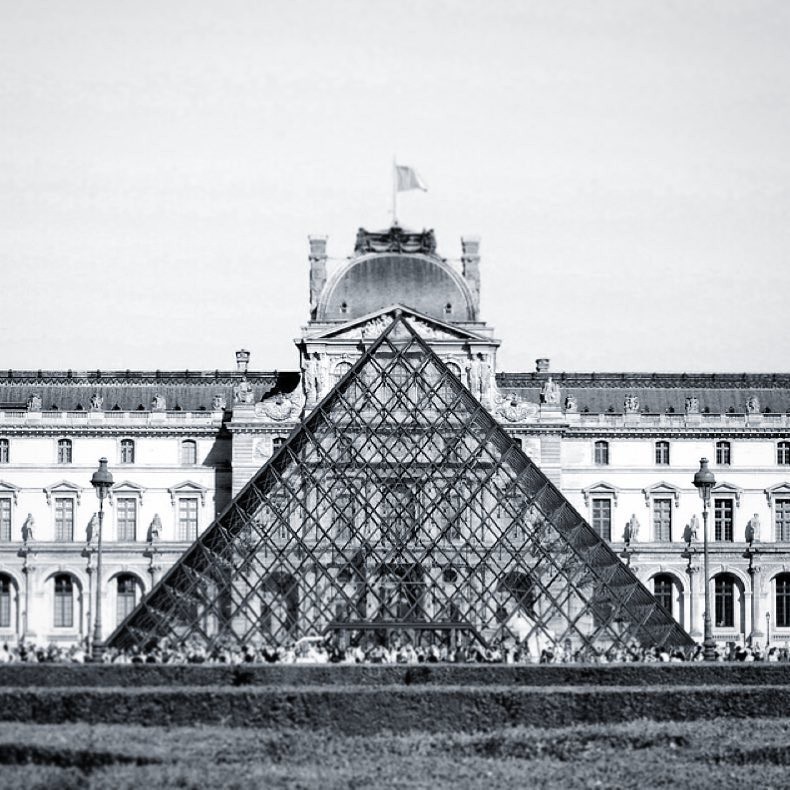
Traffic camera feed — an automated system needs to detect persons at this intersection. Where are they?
[0,634,471,664]
[90,512,98,537]
[540,644,609,663]
[762,641,790,661]
[317,354,328,393]
[469,636,536,663]
[151,513,162,528]
[543,377,556,402]
[714,642,764,662]
[235,377,252,402]
[750,513,760,533]
[691,513,700,539]
[25,513,34,540]
[481,352,494,393]
[301,353,316,399]
[608,641,670,663]
[630,512,640,538]
[668,642,704,662]
[469,354,481,393]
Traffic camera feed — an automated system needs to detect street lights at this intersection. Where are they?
[690,457,717,661]
[88,457,114,658]
[766,611,770,648]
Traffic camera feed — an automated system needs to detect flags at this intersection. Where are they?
[395,166,428,191]
